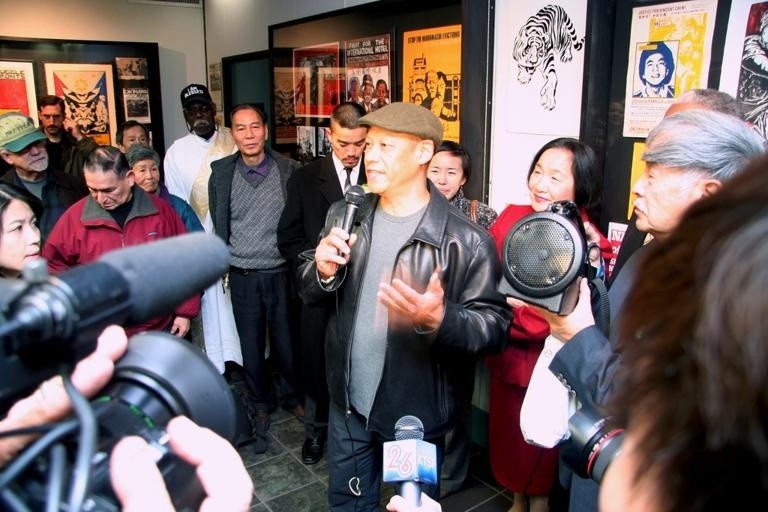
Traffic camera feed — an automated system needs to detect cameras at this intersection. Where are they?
[560,402,630,485]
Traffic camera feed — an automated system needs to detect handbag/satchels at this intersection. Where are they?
[519,334,580,450]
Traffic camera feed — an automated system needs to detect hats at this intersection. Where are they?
[358,102,443,152]
[361,75,374,87]
[180,84,212,108]
[0,113,48,152]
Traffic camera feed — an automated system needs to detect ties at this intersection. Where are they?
[343,167,352,191]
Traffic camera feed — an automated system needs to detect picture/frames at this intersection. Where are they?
[0,56,40,129]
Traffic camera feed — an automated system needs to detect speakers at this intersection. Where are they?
[496,203,598,317]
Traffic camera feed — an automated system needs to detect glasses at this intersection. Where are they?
[188,103,209,115]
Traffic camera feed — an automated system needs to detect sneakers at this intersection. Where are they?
[292,404,305,422]
[257,409,270,429]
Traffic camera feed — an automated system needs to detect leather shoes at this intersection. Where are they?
[302,425,325,465]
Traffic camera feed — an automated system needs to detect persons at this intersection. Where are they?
[116,58,147,77]
[435,72,457,121]
[417,71,439,110]
[348,73,391,115]
[633,41,675,100]
[1,84,768,511]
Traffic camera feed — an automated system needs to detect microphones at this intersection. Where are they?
[335,184,367,272]
[394,415,425,509]
